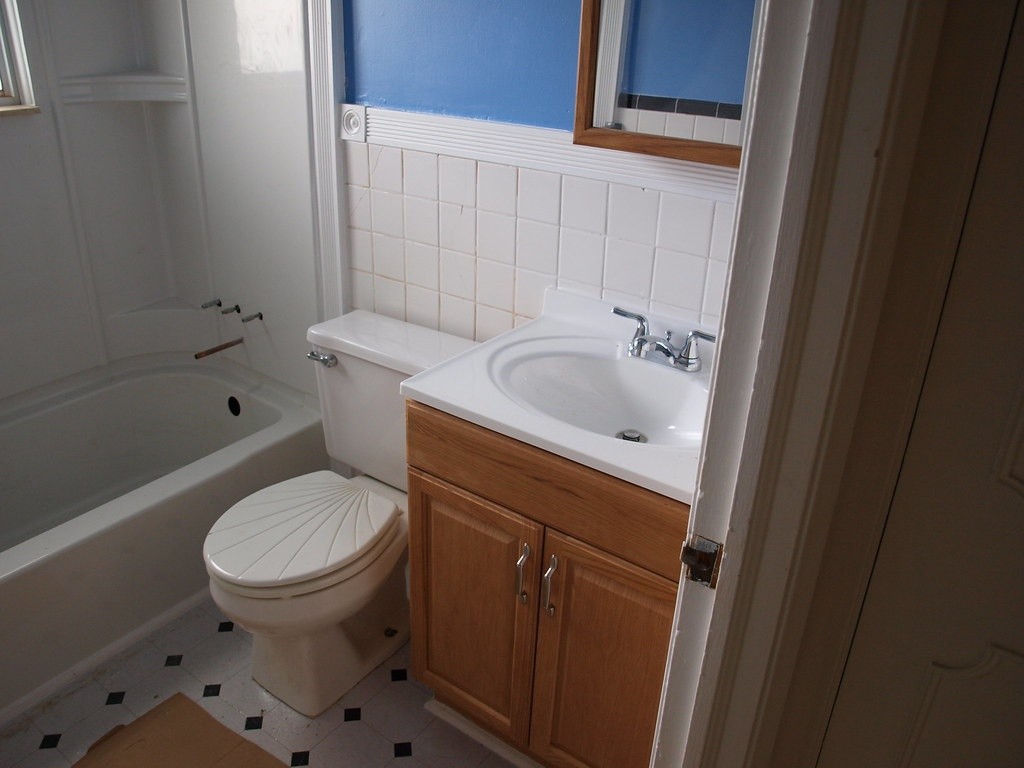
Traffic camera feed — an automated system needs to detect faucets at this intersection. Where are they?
[609,306,721,374]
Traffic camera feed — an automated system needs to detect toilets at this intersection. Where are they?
[202,308,484,720]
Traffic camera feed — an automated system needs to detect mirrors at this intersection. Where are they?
[573,0,756,170]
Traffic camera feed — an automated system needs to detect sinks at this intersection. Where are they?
[485,331,709,447]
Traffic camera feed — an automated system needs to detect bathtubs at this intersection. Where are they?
[0,348,330,738]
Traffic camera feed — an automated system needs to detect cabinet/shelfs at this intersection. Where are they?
[405,399,690,768]
